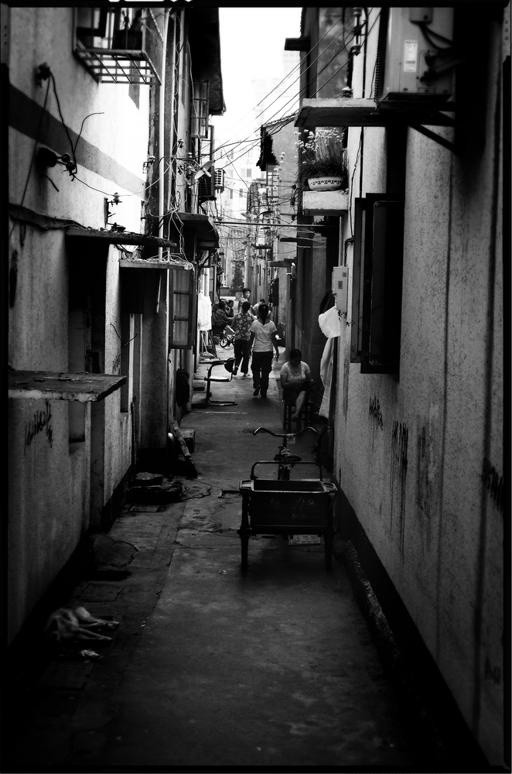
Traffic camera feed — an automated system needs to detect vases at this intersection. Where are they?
[307,175,344,190]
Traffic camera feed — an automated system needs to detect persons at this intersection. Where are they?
[248,304,279,399]
[280,348,316,420]
[211,288,265,377]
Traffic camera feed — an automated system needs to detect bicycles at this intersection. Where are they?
[220,328,236,348]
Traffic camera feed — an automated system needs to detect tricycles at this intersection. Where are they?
[236,424,340,564]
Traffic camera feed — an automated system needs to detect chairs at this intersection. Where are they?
[276,376,318,433]
[203,357,238,407]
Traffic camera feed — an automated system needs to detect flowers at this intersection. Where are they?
[293,126,348,186]
